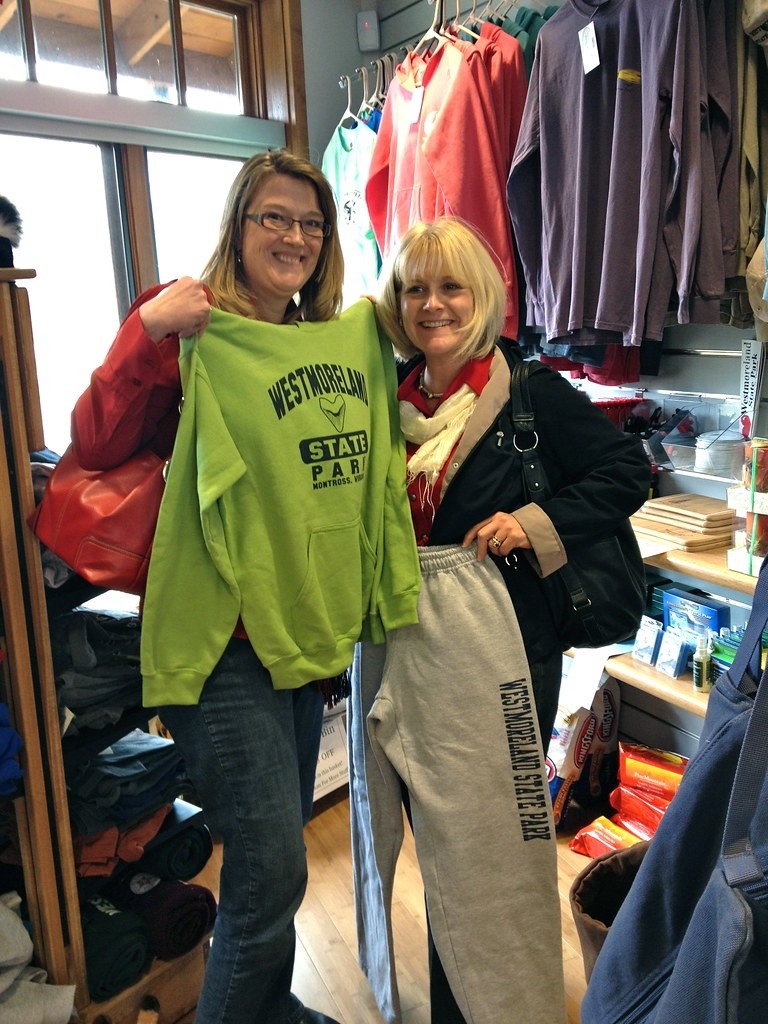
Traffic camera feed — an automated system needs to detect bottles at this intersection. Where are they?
[693,636,711,691]
[720,625,729,636]
[649,407,662,425]
[638,420,667,439]
[733,626,742,632]
[707,632,718,654]
[745,620,748,631]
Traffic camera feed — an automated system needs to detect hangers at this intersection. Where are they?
[330,0,546,128]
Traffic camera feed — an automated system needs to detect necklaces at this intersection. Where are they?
[418,370,444,398]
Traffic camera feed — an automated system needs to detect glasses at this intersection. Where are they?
[243,210,335,238]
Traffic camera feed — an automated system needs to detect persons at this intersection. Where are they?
[373,212,653,1024]
[69,151,523,1023]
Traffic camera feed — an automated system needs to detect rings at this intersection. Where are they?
[490,536,501,548]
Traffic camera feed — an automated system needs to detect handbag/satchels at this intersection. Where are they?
[33,277,173,595]
[514,359,646,648]
[580,561,766,1022]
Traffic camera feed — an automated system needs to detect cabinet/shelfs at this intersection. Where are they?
[29,469,213,1024]
[572,381,744,485]
[564,544,758,720]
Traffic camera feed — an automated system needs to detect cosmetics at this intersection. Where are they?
[632,588,768,693]
